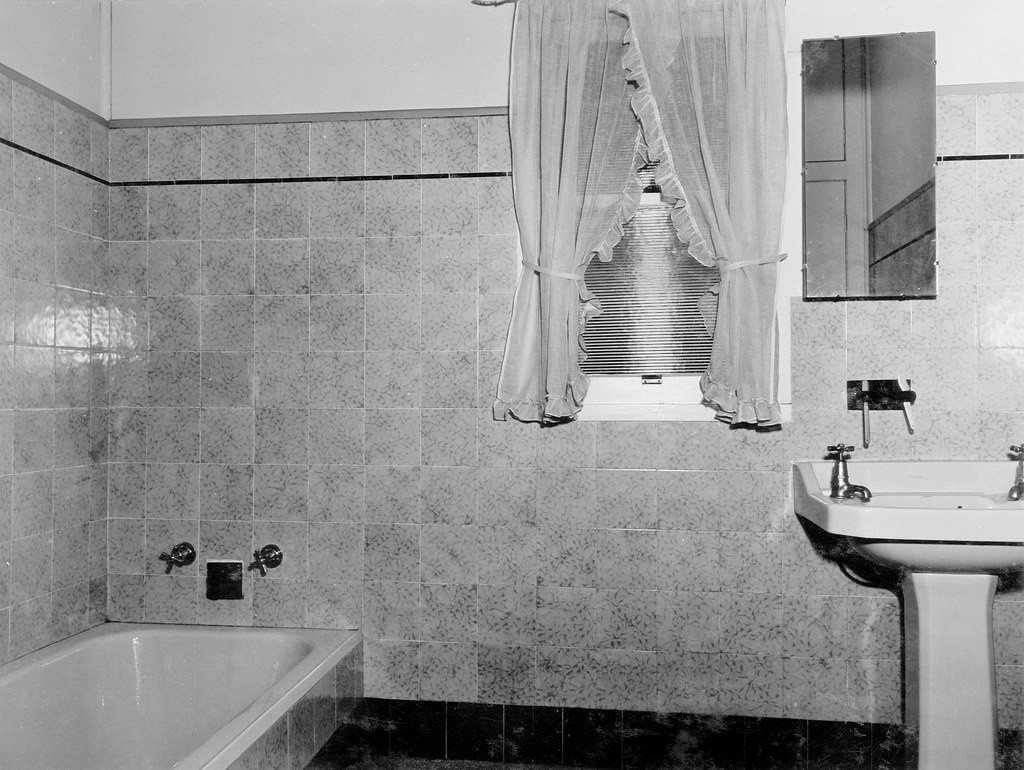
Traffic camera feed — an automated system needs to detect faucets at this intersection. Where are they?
[1008,444,1024,499]
[827,443,872,499]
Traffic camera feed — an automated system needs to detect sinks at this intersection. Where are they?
[0,623,364,770]
[794,461,1024,572]
